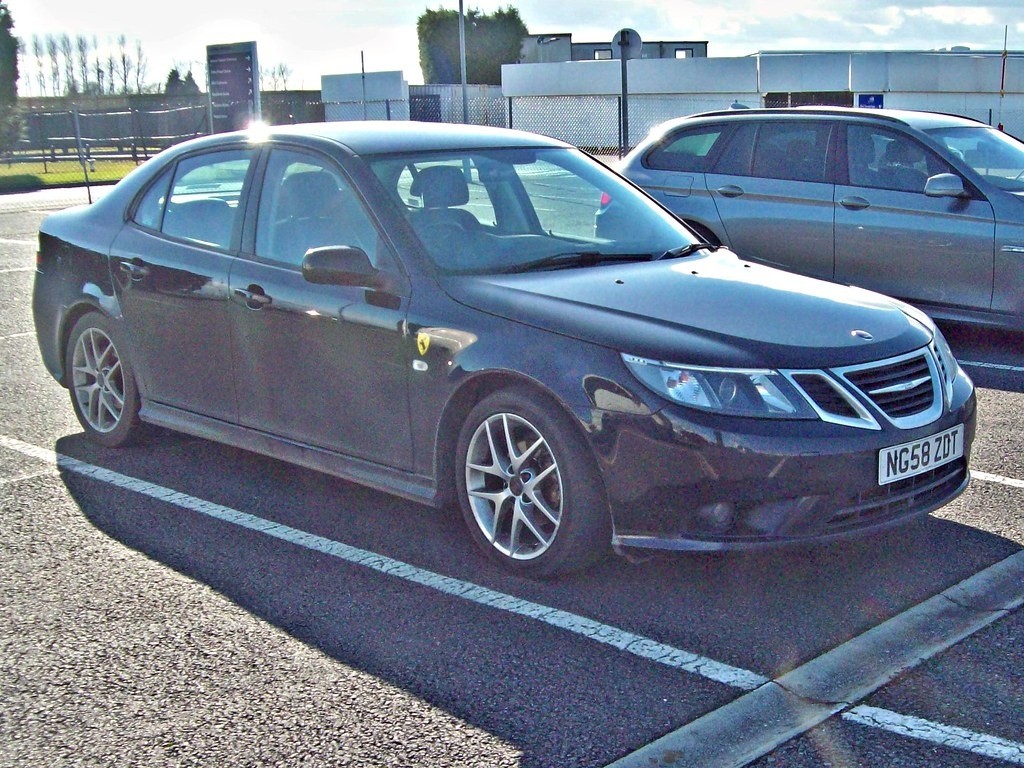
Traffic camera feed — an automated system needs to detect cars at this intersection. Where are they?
[594,104,1024,344]
[32,119,979,583]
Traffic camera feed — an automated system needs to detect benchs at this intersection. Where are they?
[174,198,371,245]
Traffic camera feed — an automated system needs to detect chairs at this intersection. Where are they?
[848,133,882,186]
[885,140,930,188]
[274,172,344,266]
[408,164,481,246]
